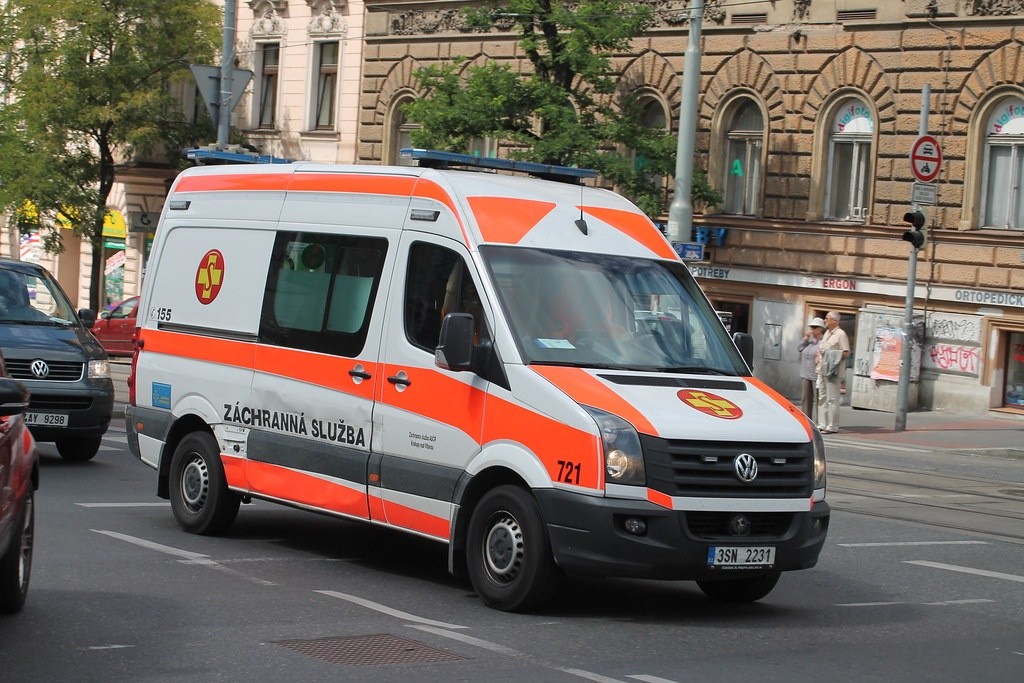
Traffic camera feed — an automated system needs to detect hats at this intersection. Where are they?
[806,317,825,329]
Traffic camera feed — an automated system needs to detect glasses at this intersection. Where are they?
[810,326,819,330]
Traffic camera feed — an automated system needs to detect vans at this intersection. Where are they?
[120,135,839,616]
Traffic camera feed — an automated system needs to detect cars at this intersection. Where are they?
[0,376,44,621]
[0,257,116,467]
[84,289,147,356]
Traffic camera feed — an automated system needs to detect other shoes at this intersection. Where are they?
[818,426,839,434]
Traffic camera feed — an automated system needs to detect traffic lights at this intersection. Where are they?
[898,209,931,251]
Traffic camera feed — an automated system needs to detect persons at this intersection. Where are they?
[798,317,826,426]
[562,292,628,345]
[814,310,850,433]
